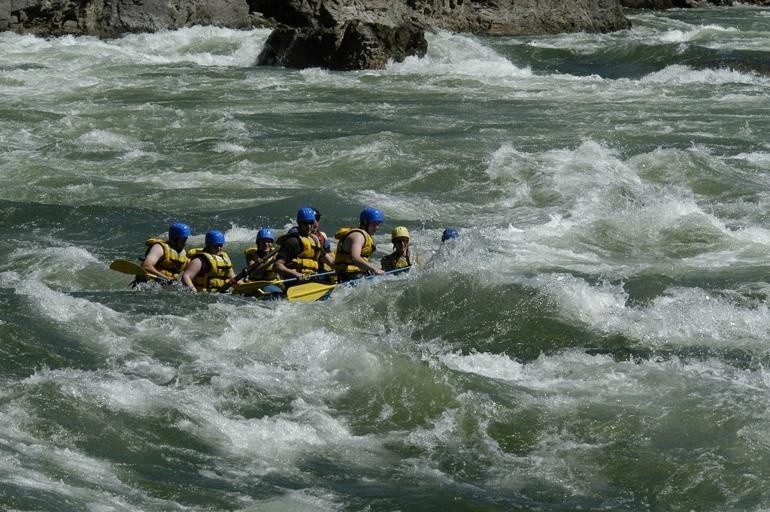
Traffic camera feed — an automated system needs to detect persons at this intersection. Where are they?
[271,206,337,301]
[180,230,245,298]
[380,225,414,275]
[128,223,190,292]
[333,206,386,284]
[275,205,338,284]
[441,228,459,243]
[244,228,287,300]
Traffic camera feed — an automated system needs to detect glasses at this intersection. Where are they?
[315,216,320,223]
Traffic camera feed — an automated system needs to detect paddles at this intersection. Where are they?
[231,270,337,295]
[287,266,412,304]
[110,259,169,283]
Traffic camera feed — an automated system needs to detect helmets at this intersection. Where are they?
[297,208,315,226]
[313,209,321,218]
[205,231,224,248]
[391,226,409,242]
[256,230,274,244]
[442,229,458,240]
[168,223,191,240]
[360,207,384,224]
